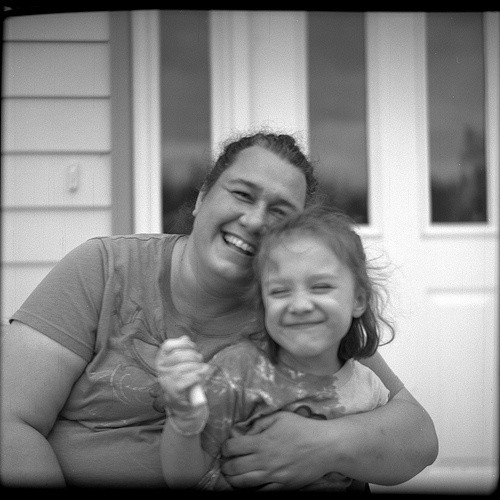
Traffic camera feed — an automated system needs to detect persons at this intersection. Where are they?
[154,204,396,493]
[0,129,440,489]
